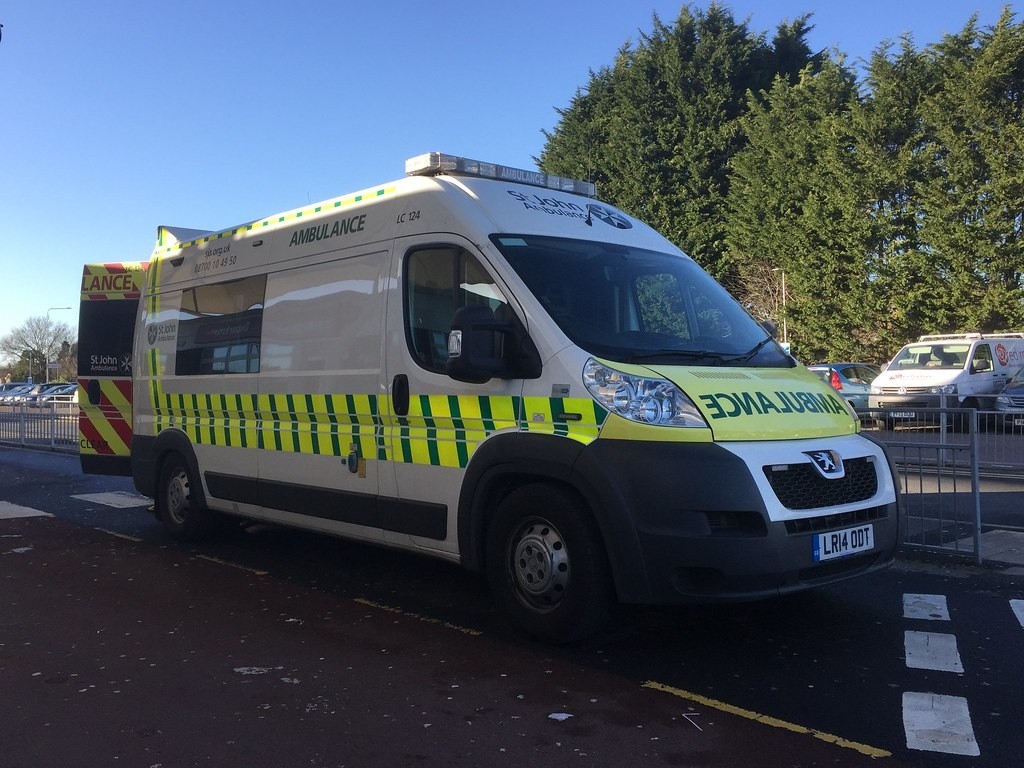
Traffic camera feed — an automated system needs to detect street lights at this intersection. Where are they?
[771,268,788,343]
[26,353,32,377]
[46,307,72,383]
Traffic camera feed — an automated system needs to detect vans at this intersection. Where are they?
[868,332,1024,431]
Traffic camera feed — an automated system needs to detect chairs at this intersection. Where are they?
[450,304,495,359]
[926,348,942,365]
[565,288,611,344]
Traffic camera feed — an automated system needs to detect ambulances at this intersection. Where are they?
[78,152,901,645]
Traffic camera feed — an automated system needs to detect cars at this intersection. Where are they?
[995,366,1024,434]
[804,363,882,428]
[0,382,78,407]
